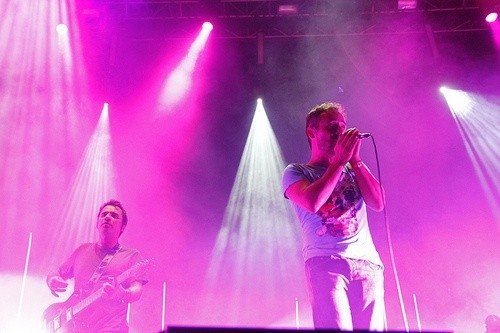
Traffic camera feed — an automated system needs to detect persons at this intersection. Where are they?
[46,199,148,333]
[283,102,388,331]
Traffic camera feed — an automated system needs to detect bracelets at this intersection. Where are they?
[119,290,128,304]
[352,160,364,168]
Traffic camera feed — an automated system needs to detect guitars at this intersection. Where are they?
[41,257,158,333]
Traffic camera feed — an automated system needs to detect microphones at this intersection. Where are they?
[357,133,371,139]
